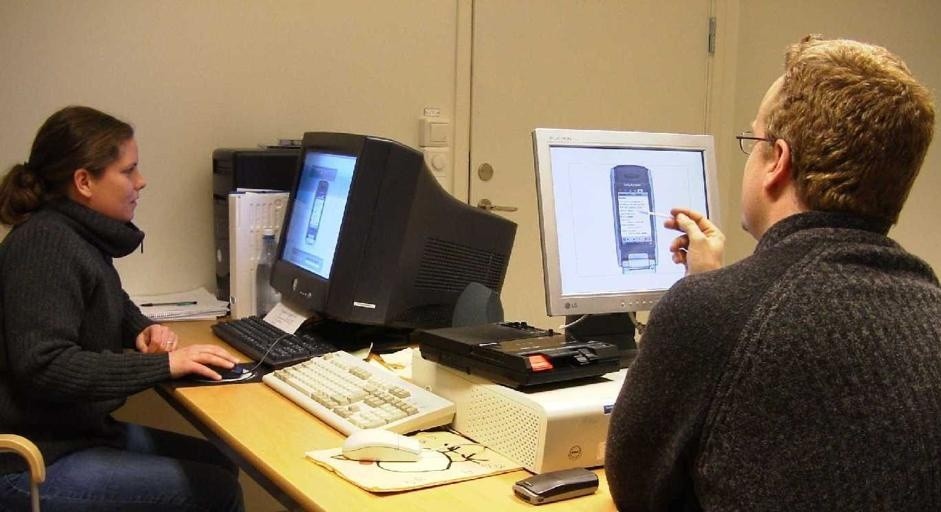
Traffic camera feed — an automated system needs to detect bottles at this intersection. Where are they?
[256,228,281,317]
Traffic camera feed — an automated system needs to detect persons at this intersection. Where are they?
[604,32,940,512]
[0,105,246,512]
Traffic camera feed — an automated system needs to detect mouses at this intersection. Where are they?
[206,363,242,380]
[341,428,421,463]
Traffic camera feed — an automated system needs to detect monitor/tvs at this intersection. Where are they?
[269,131,519,351]
[530,126,719,367]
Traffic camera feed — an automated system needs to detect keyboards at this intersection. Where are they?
[261,350,455,439]
[209,315,338,372]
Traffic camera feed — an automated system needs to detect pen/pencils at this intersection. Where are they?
[140,301,197,306]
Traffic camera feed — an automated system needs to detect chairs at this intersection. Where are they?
[1,432,45,511]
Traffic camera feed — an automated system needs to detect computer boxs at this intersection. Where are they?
[211,146,305,308]
[409,348,629,476]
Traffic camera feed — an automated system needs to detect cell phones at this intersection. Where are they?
[513,466,599,507]
[304,181,330,243]
[610,163,660,274]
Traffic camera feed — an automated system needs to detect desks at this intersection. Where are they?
[131,320,621,512]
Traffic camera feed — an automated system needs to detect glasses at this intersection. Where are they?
[736,131,769,155]
